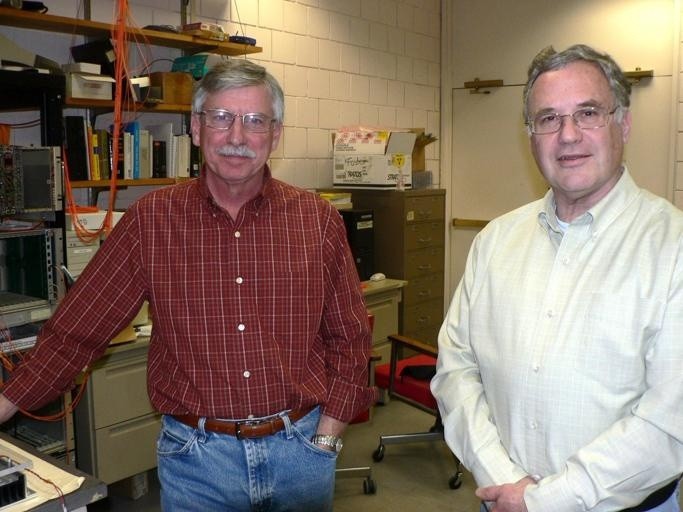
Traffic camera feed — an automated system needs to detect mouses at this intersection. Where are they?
[370,272,386,282]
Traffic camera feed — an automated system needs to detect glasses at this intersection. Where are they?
[523,107,622,135]
[194,108,278,134]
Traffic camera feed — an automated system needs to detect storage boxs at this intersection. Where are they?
[326,125,439,191]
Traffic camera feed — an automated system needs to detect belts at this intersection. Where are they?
[609,474,681,511]
[168,407,315,437]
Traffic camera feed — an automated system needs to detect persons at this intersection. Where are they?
[426,41,682,511]
[0,60,374,508]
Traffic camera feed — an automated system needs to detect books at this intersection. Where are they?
[64,115,200,182]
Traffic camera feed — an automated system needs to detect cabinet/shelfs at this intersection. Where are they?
[349,186,450,360]
[2,2,411,503]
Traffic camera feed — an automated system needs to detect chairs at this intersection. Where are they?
[372,334,468,492]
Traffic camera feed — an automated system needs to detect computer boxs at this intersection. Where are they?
[337,208,375,282]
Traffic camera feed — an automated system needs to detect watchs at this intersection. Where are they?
[310,434,343,453]
